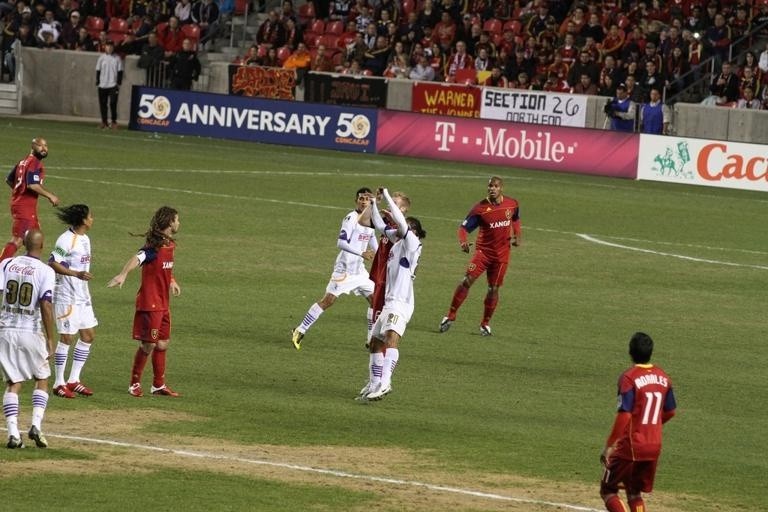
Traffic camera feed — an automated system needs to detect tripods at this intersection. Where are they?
[602,114,618,130]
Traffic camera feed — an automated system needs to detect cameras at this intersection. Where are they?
[603,99,620,119]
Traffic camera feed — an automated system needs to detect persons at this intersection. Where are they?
[95,40,124,129]
[232,1,768,110]
[599,332,676,512]
[95,30,108,52]
[291,188,382,350]
[0,228,56,449]
[47,204,99,398]
[603,83,670,135]
[439,176,522,335]
[105,207,182,397]
[0,136,61,264]
[0,0,95,84]
[125,1,231,91]
[359,187,424,400]
[368,192,411,355]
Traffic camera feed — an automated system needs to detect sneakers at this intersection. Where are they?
[52,384,76,398]
[7,435,24,448]
[66,382,93,394]
[367,382,392,399]
[291,328,304,349]
[480,324,491,336]
[439,316,452,332]
[128,383,144,397]
[354,391,381,401]
[150,385,179,396]
[29,426,48,448]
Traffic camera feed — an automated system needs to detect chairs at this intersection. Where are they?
[481,8,526,35]
[299,0,424,73]
[83,16,200,49]
